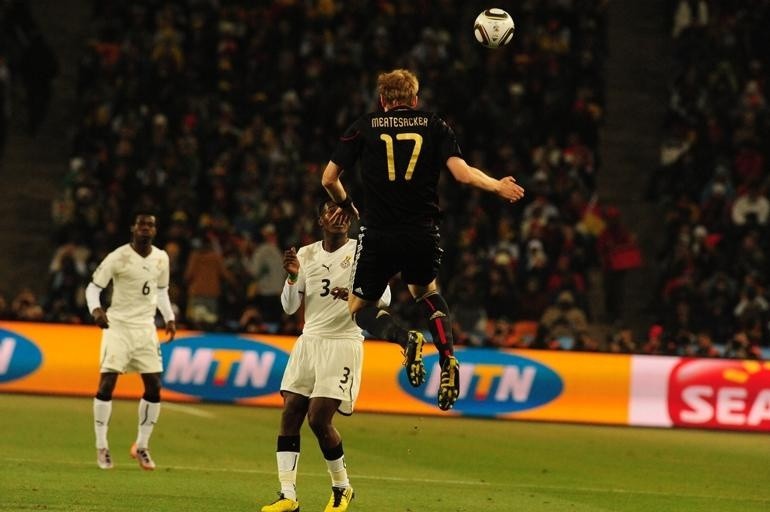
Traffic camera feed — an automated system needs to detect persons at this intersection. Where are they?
[85,207,176,469]
[321,67,527,411]
[257,194,393,511]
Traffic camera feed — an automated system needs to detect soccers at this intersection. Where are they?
[473,4,515,49]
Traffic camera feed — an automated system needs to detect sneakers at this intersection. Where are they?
[403,330,427,388]
[323,485,355,512]
[437,355,460,411]
[261,492,300,512]
[97,447,114,470]
[130,442,156,470]
